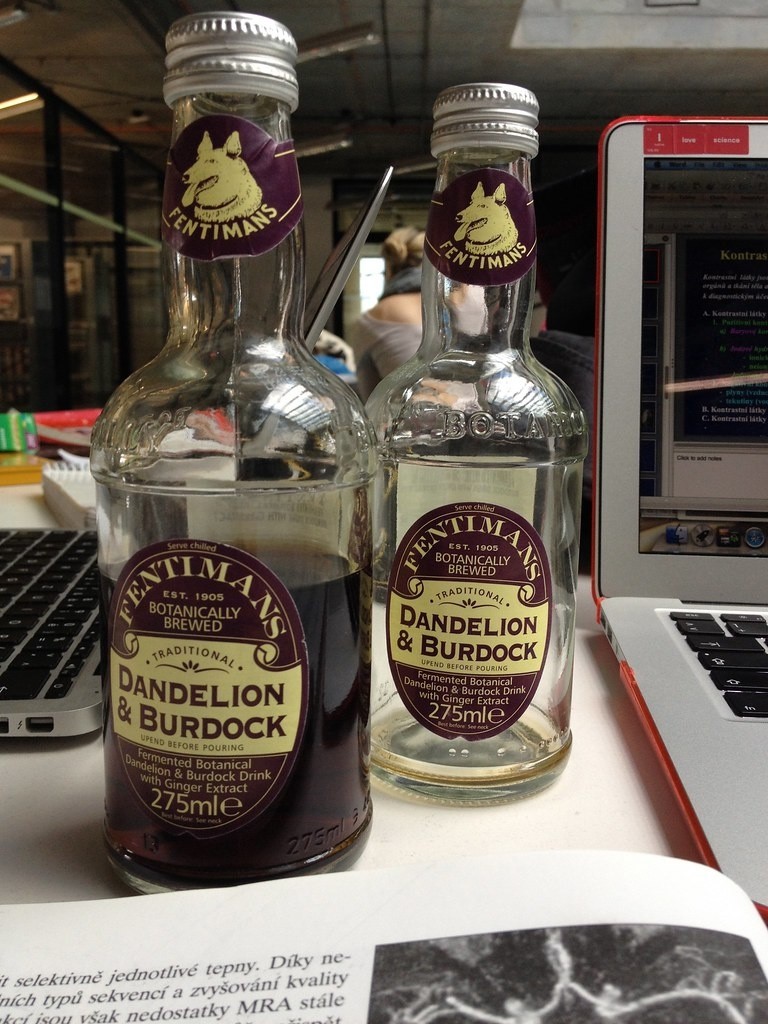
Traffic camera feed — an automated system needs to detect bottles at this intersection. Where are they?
[87,7,381,896]
[363,81,594,806]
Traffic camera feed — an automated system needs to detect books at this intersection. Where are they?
[0,847,768,1024]
[40,462,98,530]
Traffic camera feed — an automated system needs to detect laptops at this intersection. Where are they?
[595,114,768,916]
[0,160,396,739]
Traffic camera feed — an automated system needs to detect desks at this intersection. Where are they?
[0,602,696,904]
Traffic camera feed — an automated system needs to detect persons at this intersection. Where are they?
[357,223,488,402]
